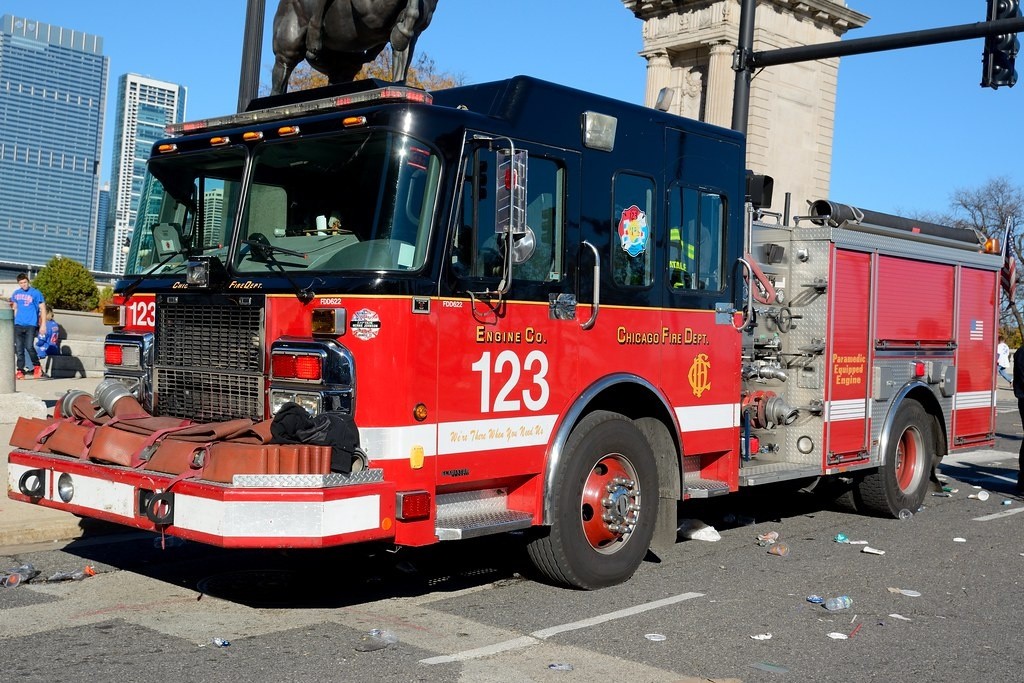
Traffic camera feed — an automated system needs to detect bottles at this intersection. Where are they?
[826,596,854,610]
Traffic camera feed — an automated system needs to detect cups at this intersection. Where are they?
[768,542,790,556]
[899,509,913,522]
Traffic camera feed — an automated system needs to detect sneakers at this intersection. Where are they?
[15,371,25,380]
[33,366,42,379]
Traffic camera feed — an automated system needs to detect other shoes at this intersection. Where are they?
[1017,493,1024,499]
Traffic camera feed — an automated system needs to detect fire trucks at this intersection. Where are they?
[8,75,1005,592]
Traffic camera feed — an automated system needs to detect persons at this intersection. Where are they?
[1013,342,1024,499]
[11,273,47,379]
[997,336,1013,388]
[25,305,59,375]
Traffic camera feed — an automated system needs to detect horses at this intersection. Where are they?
[269,0,440,97]
